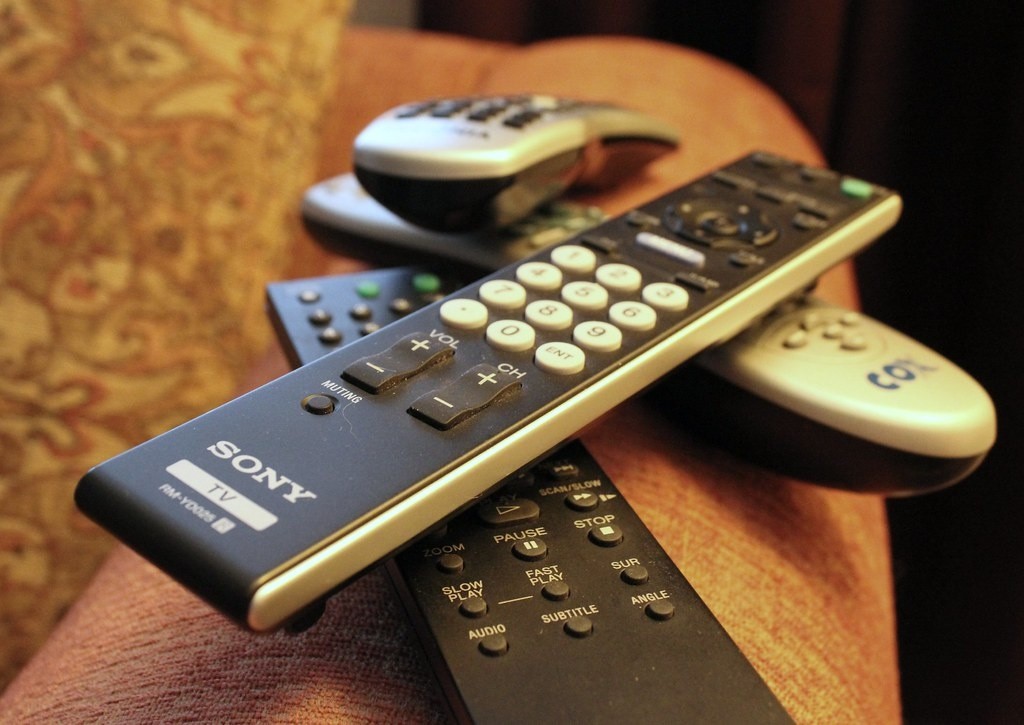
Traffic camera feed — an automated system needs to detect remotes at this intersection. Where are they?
[73,95,999,725]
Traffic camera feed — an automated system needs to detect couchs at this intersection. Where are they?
[0,0,903,725]
